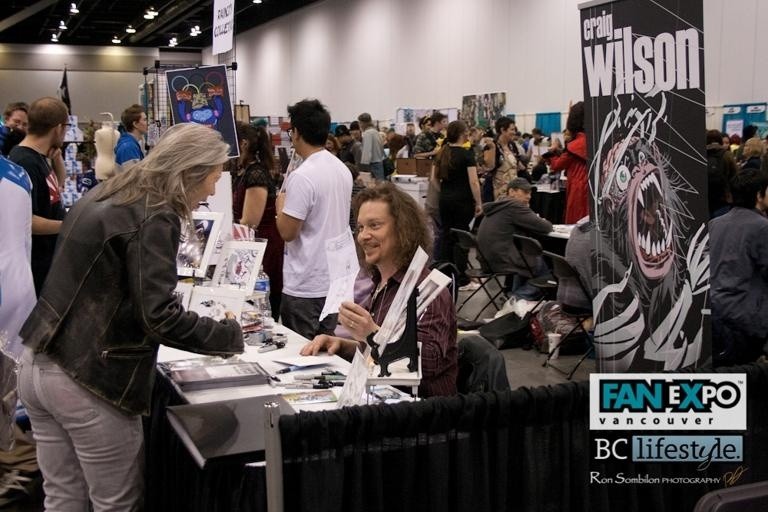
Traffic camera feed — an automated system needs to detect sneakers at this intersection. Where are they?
[458,281,482,291]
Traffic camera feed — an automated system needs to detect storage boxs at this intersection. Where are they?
[396,180,431,210]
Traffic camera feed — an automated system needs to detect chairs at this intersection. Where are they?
[541,250,593,380]
[512,233,557,313]
[450,228,518,321]
[457,334,510,395]
[694,482,768,512]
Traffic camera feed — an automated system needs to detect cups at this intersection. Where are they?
[547,333,561,360]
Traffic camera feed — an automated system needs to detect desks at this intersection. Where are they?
[545,224,576,239]
[143,280,470,512]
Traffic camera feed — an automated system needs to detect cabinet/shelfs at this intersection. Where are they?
[59,140,95,209]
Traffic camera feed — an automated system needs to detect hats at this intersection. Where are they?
[507,177,537,194]
[334,125,351,137]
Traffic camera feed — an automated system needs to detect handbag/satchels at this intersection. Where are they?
[383,158,395,177]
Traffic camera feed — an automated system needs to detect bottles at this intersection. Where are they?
[252,265,272,320]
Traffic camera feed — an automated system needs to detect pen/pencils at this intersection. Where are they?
[258,341,285,353]
[285,372,347,390]
[277,365,309,373]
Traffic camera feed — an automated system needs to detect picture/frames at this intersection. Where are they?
[173,281,194,310]
[176,211,225,278]
[211,241,267,295]
[188,286,246,323]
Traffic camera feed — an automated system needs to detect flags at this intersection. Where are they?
[56,69,71,115]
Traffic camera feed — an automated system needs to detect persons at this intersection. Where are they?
[0,94,768,511]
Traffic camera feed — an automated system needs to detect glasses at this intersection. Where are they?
[287,128,293,137]
[63,122,73,130]
